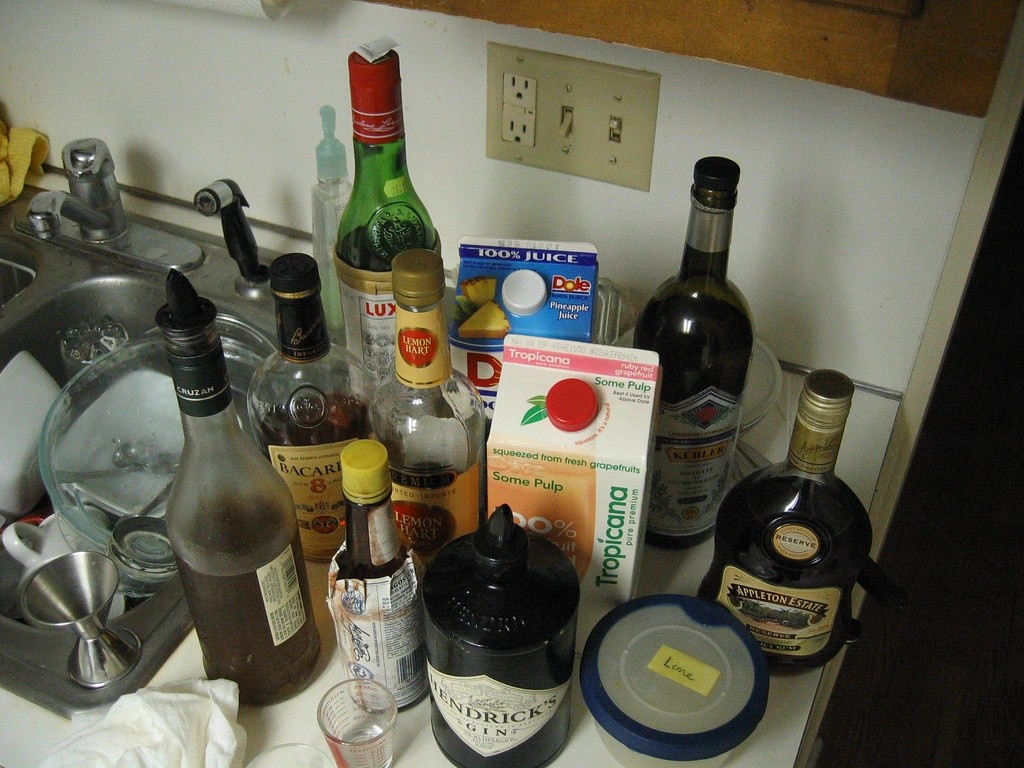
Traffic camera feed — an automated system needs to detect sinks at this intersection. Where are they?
[0,183,103,336]
[0,224,348,721]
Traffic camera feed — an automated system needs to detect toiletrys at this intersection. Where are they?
[311,105,353,333]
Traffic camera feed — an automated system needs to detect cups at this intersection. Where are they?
[316,677,397,768]
[1,506,125,620]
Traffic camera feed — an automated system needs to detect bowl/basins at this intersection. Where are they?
[578,594,770,768]
[37,342,268,596]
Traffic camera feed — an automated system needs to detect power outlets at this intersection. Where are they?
[484,41,661,192]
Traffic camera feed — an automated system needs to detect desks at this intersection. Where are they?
[0,165,903,768]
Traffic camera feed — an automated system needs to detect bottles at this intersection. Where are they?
[310,104,354,336]
[155,268,322,707]
[246,252,370,561]
[422,504,579,768]
[628,156,753,550]
[699,368,872,671]
[334,46,441,401]
[326,439,429,714]
[369,249,487,570]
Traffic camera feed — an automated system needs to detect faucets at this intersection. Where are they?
[26,137,129,244]
